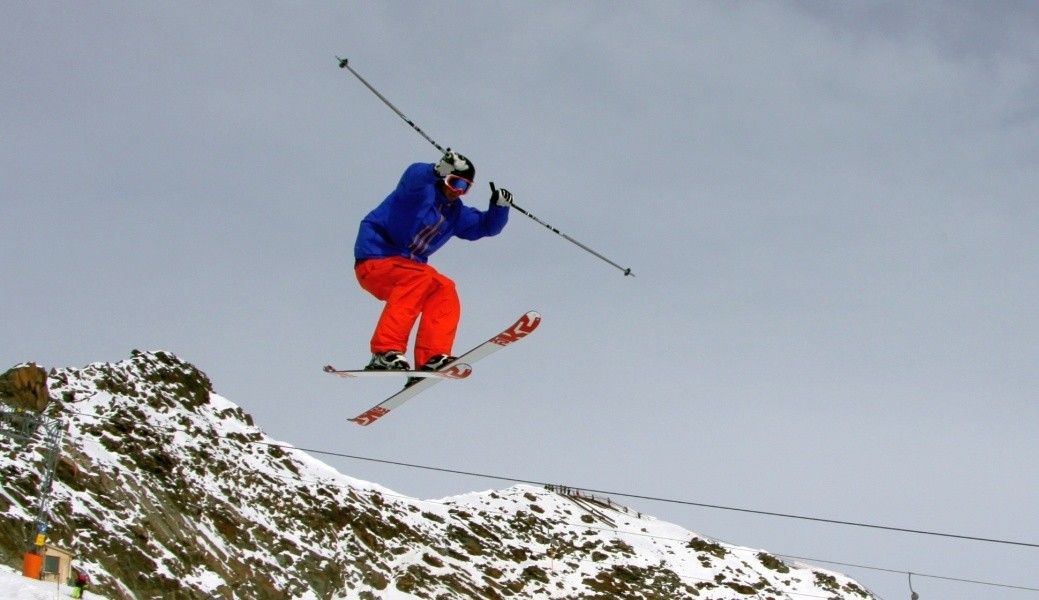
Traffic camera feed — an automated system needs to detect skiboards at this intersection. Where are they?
[322,310,542,426]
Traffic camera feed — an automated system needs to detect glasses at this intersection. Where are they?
[444,174,473,195]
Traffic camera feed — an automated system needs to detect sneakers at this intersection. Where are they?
[414,354,457,381]
[364,350,410,371]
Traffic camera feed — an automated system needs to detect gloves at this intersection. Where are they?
[490,188,513,207]
[434,147,469,178]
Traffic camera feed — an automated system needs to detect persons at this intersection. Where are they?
[352,149,513,374]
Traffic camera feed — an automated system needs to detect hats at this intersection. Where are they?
[451,153,475,184]
